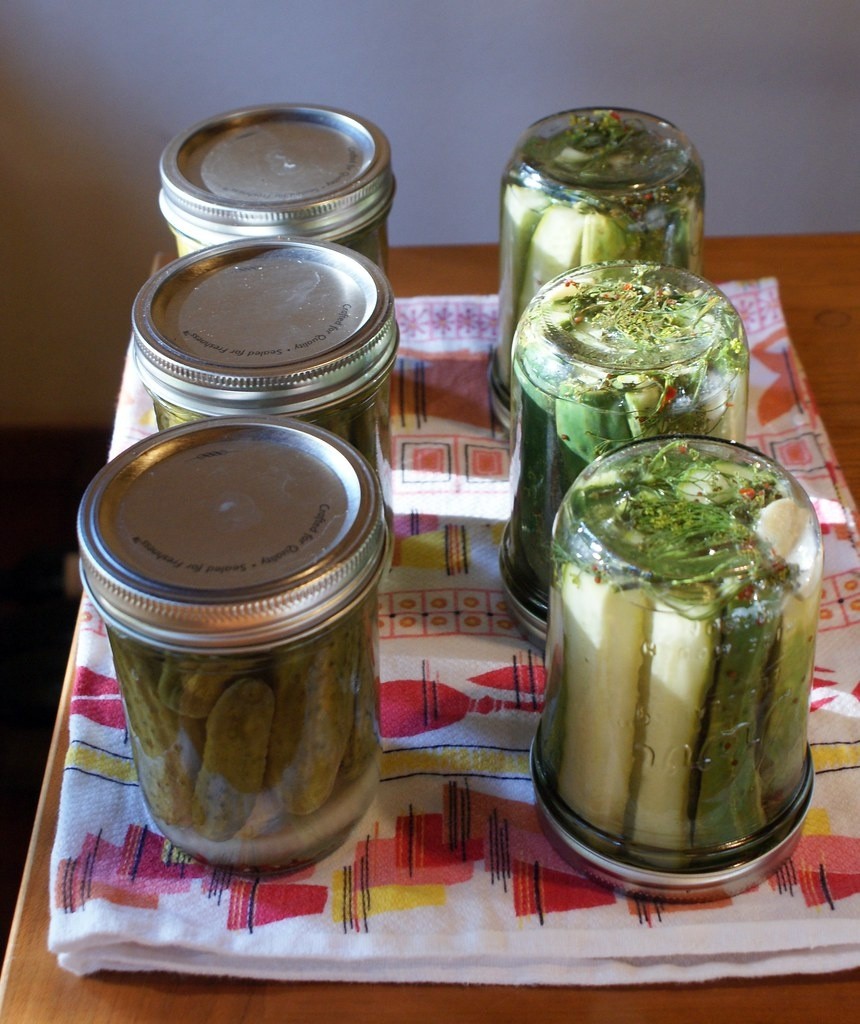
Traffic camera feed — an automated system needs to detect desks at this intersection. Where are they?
[0,229,860,1023]
